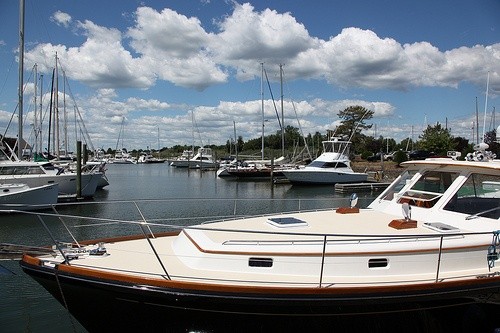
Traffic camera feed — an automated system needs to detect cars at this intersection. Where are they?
[354,150,435,162]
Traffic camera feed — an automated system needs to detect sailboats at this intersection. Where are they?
[215,62,312,177]
[0,0,138,212]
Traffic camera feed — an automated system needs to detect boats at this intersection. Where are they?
[269,136,368,184]
[166,104,229,168]
[137,152,164,163]
[18,158,500,333]
